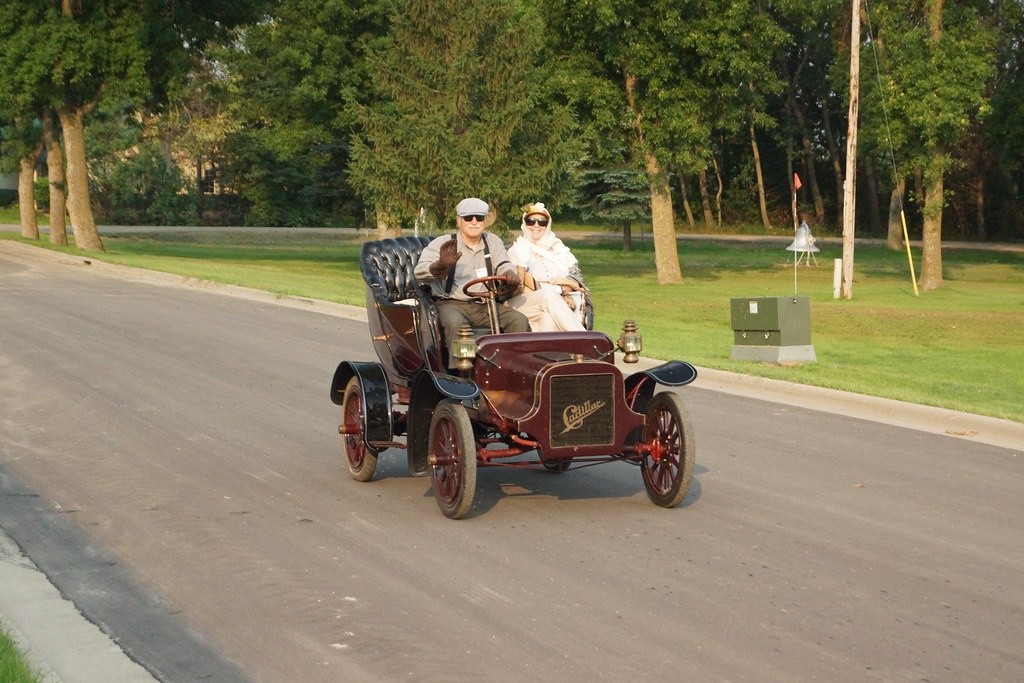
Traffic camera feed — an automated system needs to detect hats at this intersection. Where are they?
[456,198,489,216]
[520,202,550,219]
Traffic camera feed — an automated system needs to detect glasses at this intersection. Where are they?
[463,215,485,221]
[526,217,548,227]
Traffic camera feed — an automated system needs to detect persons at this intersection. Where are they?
[507,202,588,332]
[413,197,529,373]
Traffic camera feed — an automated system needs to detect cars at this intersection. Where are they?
[329,234,699,521]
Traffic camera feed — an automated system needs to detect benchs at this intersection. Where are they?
[359,235,594,389]
